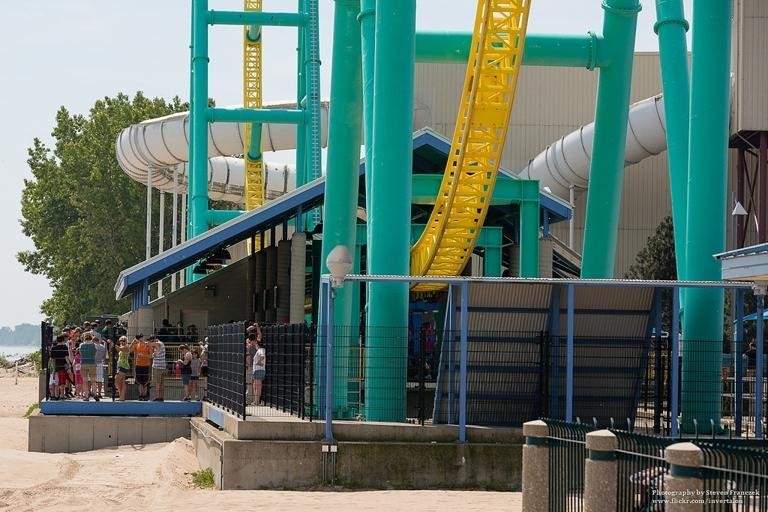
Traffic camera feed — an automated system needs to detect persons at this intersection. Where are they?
[200,336,209,400]
[155,318,175,344]
[742,342,757,378]
[184,322,198,342]
[242,322,262,403]
[189,349,200,401]
[176,343,193,401]
[248,335,267,406]
[48,315,167,402]
[172,321,187,343]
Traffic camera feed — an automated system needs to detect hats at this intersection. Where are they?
[147,336,154,341]
[136,333,142,337]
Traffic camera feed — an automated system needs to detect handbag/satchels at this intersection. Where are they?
[75,363,82,370]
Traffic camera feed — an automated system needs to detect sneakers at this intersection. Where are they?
[139,396,164,401]
[183,397,208,401]
[49,392,102,401]
[245,400,260,405]
